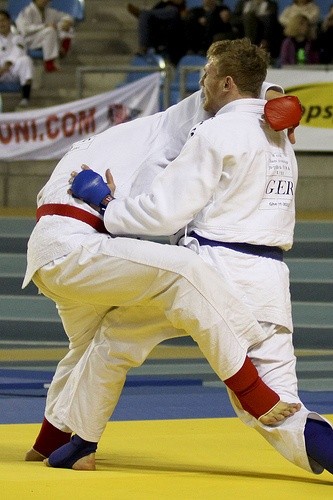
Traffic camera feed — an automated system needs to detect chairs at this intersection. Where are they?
[1,0,332,113]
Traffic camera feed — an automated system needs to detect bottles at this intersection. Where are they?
[297,49,305,65]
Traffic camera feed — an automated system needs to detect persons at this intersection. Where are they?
[21,82,302,461]
[0,9,34,112]
[127,0,333,67]
[43,38,333,474]
[14,0,76,74]
